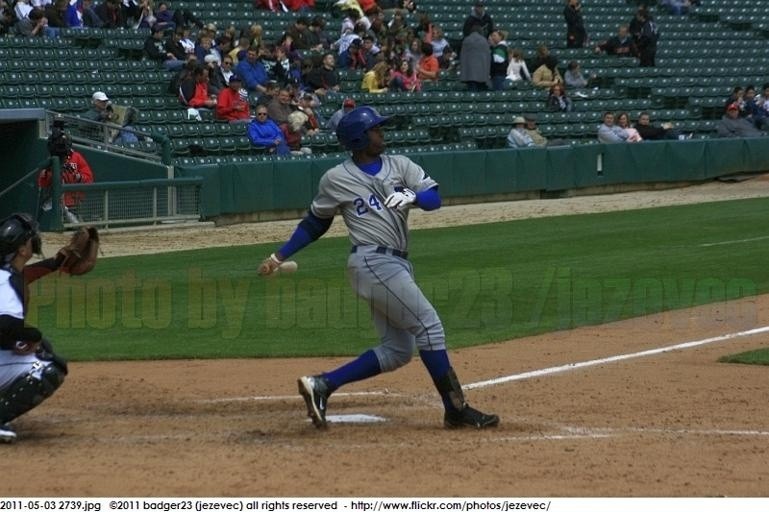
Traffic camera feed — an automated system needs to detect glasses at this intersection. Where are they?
[224,62,233,65]
[258,112,267,116]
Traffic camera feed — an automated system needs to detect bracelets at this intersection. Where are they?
[270,253,282,264]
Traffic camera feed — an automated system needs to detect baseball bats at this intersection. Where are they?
[260,262,296,272]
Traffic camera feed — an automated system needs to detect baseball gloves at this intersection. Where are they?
[57,227,98,276]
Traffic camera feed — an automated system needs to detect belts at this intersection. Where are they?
[351,244,408,261]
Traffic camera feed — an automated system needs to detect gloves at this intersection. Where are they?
[260,253,283,276]
[384,187,417,209]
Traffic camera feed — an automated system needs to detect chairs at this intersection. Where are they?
[0,0,769,167]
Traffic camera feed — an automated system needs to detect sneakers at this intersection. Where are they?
[296,375,332,432]
[443,402,501,431]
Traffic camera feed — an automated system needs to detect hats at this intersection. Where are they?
[727,103,738,111]
[299,92,313,101]
[524,114,538,121]
[92,91,110,102]
[513,116,527,124]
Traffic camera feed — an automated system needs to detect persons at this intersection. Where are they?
[255,105,499,429]
[37,132,93,225]
[0,212,68,443]
[1,0,769,152]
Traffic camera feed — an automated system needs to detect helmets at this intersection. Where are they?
[336,105,393,150]
[1,212,40,265]
